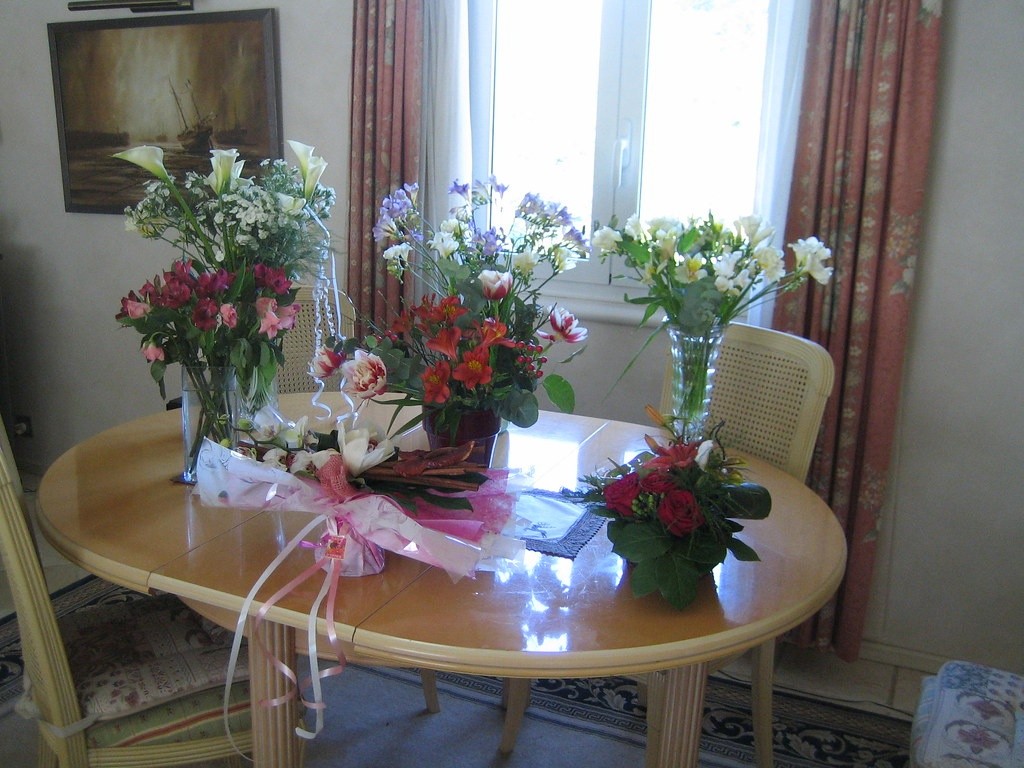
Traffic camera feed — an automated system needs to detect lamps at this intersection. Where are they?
[68,0,193,13]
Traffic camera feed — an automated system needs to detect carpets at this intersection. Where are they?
[0,574,912,768]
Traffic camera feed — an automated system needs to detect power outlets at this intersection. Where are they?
[15,415,33,437]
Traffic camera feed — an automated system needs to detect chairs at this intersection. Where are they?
[908,658,1024,768]
[498,325,834,768]
[275,286,441,716]
[0,418,308,768]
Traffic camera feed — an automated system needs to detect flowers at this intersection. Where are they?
[307,269,588,439]
[191,406,535,585]
[590,206,835,403]
[559,403,772,611]
[114,258,302,401]
[370,175,591,327]
[110,138,350,293]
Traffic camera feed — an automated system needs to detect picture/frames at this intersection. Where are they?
[47,8,284,215]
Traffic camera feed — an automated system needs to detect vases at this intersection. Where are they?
[181,365,239,484]
[662,316,726,442]
[422,400,502,469]
[233,370,278,430]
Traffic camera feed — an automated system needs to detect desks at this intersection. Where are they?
[35,391,849,768]
